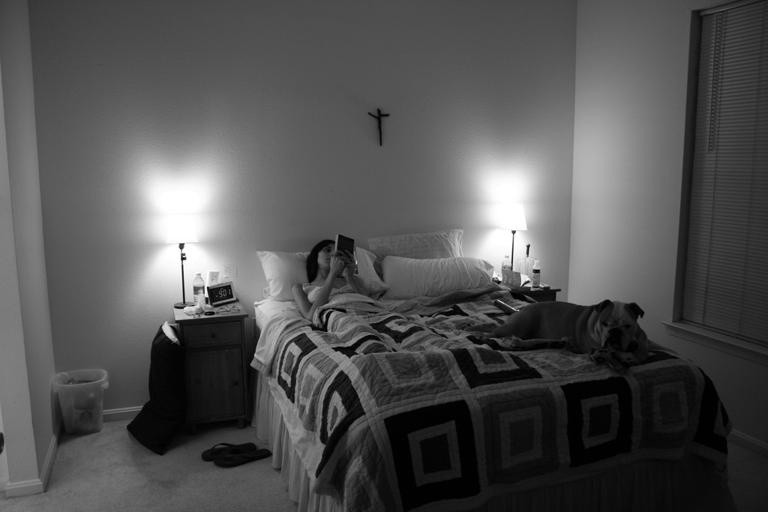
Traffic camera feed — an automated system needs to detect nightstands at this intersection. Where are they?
[173,304,248,430]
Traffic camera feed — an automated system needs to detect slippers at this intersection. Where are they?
[201,443,272,469]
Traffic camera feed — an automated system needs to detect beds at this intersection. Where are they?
[250,263,713,512]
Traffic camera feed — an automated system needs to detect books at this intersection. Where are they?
[335,234,359,274]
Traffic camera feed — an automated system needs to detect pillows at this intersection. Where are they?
[260,227,498,304]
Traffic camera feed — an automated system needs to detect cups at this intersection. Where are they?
[509,271,522,289]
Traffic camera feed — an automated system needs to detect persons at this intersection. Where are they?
[292,239,359,322]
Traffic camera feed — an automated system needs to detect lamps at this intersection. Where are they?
[505,203,528,270]
[164,216,204,308]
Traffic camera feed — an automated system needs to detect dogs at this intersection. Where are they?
[465,299,651,378]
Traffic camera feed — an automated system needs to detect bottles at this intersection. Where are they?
[531,259,542,288]
[190,273,206,312]
[500,255,513,289]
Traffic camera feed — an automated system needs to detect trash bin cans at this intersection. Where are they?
[55,369,109,435]
